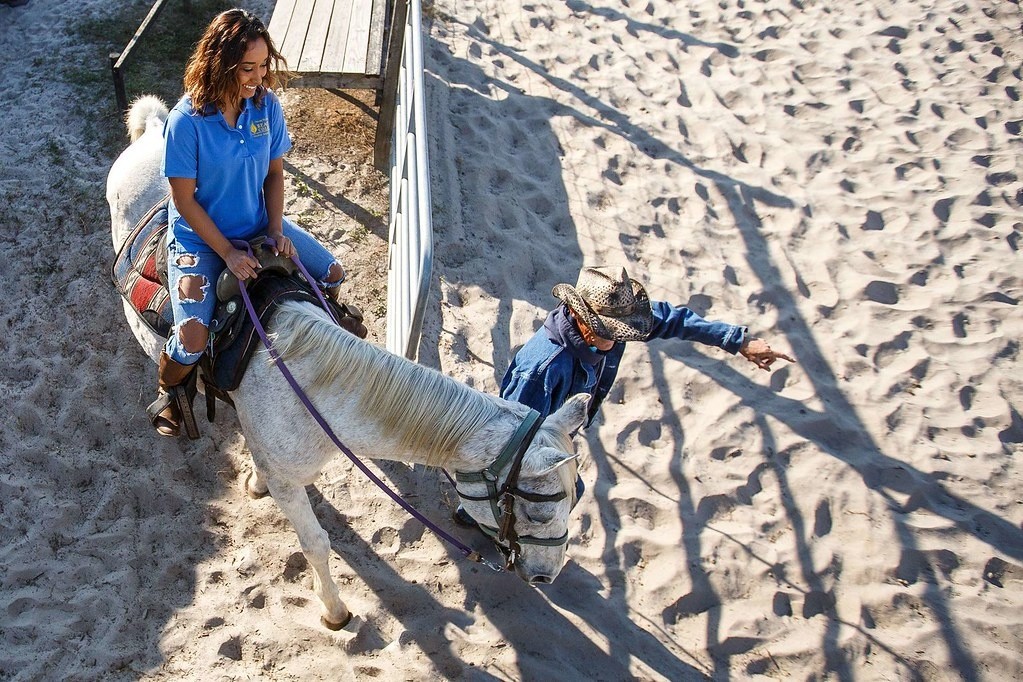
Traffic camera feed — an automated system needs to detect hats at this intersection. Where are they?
[551,265,654,342]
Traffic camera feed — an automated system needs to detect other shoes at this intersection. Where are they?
[453,510,478,528]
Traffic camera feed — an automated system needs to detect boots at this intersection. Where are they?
[154,343,198,436]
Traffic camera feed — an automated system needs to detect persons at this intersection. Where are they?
[453,266,796,530]
[154,9,368,438]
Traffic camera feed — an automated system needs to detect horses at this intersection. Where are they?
[105,94,592,631]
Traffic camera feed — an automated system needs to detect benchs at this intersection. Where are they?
[259,0,408,169]
